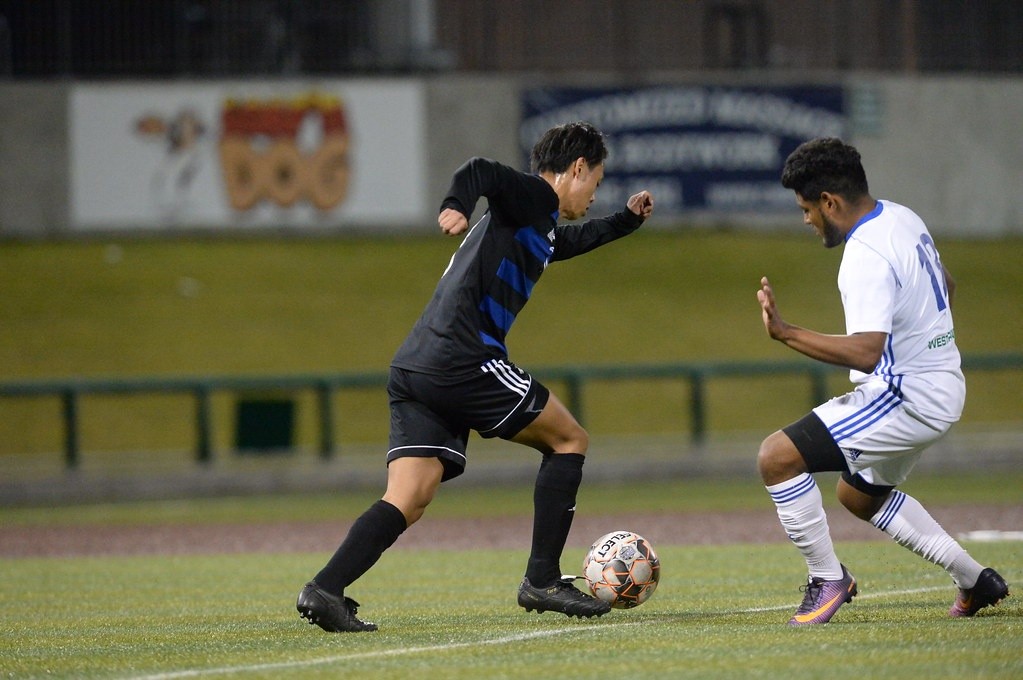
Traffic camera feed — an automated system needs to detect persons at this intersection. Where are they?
[297,123,657,631]
[755,137,1010,625]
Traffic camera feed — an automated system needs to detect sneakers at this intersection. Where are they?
[294,579,377,632]
[950,567,1009,616]
[516,574,610,619]
[787,565,860,623]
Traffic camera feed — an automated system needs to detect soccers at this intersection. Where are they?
[585,532,663,609]
